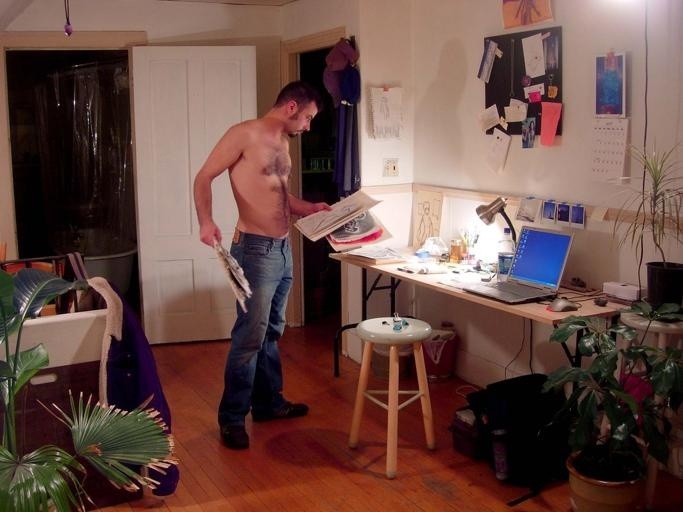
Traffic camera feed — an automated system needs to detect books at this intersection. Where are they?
[346,247,407,265]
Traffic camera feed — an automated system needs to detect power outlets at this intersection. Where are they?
[383,159,401,177]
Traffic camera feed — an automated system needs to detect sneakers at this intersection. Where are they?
[219,422,250,450]
[251,398,308,424]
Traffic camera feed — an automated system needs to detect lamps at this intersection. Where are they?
[476,198,518,252]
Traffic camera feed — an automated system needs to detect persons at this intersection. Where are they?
[194,80,333,450]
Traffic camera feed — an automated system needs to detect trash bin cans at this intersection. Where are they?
[418,329,457,382]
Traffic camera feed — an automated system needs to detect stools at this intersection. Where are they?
[351,314,436,477]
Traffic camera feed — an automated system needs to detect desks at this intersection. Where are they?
[328,245,620,436]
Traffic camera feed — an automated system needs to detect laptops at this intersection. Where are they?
[462,225,578,306]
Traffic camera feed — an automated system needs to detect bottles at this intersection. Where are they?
[450,238,462,264]
[496,228,515,282]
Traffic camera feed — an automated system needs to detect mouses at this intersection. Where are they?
[548,298,584,312]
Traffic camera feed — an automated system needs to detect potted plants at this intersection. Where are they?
[612,145,683,314]
[551,312,683,512]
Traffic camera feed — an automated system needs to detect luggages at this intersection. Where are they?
[445,373,572,487]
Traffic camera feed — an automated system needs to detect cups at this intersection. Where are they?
[449,239,461,264]
